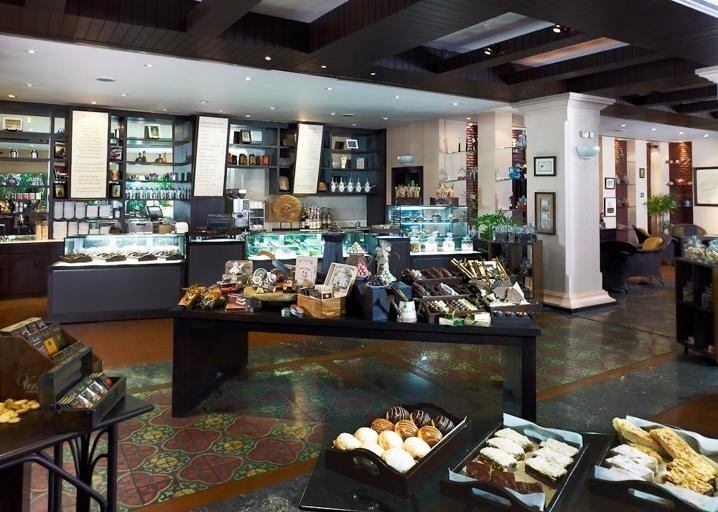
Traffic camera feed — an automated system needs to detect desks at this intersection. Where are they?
[169,294,541,422]
[0,394,155,512]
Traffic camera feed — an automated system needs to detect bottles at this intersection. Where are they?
[30,149,38,159]
[125,149,182,200]
[9,149,18,157]
[301,202,332,230]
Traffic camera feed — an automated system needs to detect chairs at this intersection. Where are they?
[600,223,706,296]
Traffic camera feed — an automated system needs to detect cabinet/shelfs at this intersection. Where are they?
[247,231,327,274]
[444,150,467,204]
[388,204,482,269]
[46,240,189,323]
[224,119,387,229]
[675,257,718,363]
[0,100,194,239]
[615,161,636,232]
[494,146,514,212]
[665,162,692,212]
[488,240,543,303]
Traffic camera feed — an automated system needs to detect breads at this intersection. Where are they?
[610,417,718,497]
[460,428,579,510]
[332,403,456,473]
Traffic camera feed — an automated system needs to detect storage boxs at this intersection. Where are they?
[0,316,94,402]
[37,346,126,435]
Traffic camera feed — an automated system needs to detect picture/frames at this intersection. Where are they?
[605,178,615,189]
[604,197,616,216]
[534,191,555,233]
[640,168,645,178]
[693,166,718,206]
[534,156,557,175]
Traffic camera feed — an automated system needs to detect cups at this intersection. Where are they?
[356,221,361,229]
[33,178,41,185]
[115,210,120,219]
